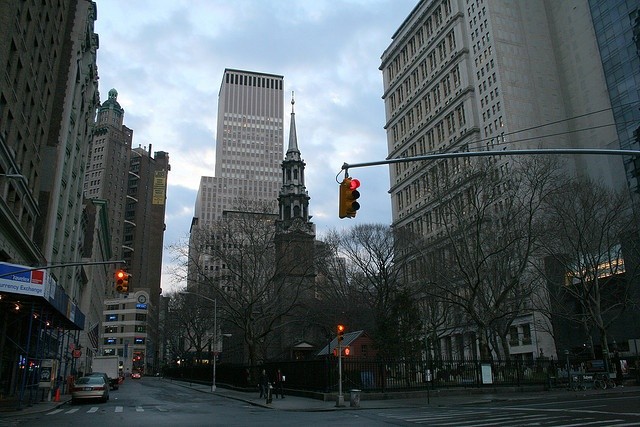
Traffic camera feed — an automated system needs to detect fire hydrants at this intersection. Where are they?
[55,388,60,401]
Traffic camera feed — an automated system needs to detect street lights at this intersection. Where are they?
[179,290,216,393]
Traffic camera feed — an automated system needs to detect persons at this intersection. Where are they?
[273,368,285,399]
[257,368,269,398]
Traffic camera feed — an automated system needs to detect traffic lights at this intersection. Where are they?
[339,176,360,218]
[345,347,350,355]
[333,348,338,356]
[337,324,344,340]
[116,271,131,294]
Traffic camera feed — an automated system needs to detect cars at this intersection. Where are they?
[72,377,109,403]
[89,372,108,381]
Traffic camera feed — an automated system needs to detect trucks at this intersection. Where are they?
[91,355,119,389]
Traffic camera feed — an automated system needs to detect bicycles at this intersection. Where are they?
[593,373,616,390]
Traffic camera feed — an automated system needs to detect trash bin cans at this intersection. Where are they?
[349,389,361,406]
